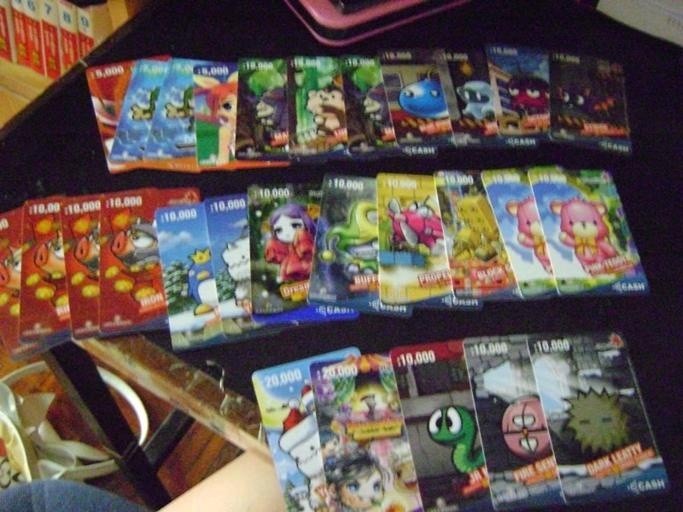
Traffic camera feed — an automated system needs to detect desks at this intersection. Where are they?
[2,2,683,510]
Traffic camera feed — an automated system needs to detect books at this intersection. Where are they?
[22,0,41,73]
[41,1,60,81]
[10,0,26,66]
[0,0,18,63]
[57,0,84,77]
[77,0,114,61]
